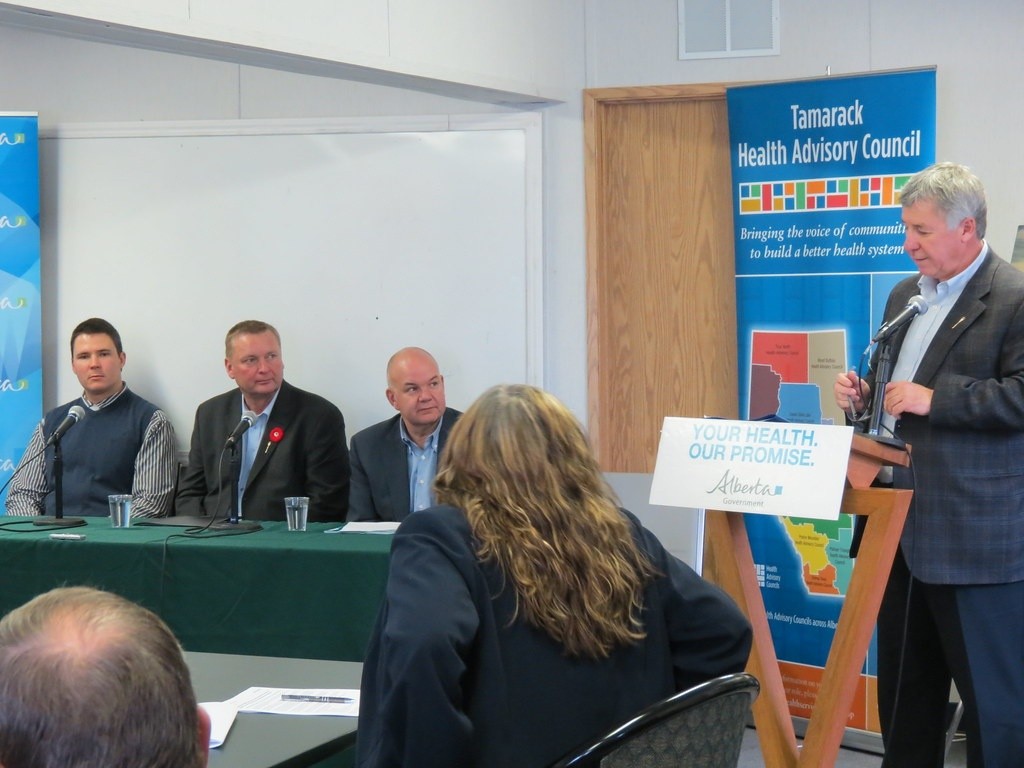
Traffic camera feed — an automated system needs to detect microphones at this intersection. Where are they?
[872,295,929,343]
[224,410,258,450]
[47,406,86,446]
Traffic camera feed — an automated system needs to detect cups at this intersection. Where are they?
[108,495,132,529]
[284,497,309,532]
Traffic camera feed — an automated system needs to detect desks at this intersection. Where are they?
[181,650,365,768]
[0,516,398,660]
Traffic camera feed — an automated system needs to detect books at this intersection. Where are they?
[324,525,402,534]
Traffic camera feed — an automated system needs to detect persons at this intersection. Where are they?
[0,587,212,768]
[345,347,463,525]
[4,318,178,519]
[174,321,352,523]
[834,162,1024,768]
[354,383,753,768]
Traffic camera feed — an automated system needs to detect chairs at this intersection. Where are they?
[555,670,761,768]
